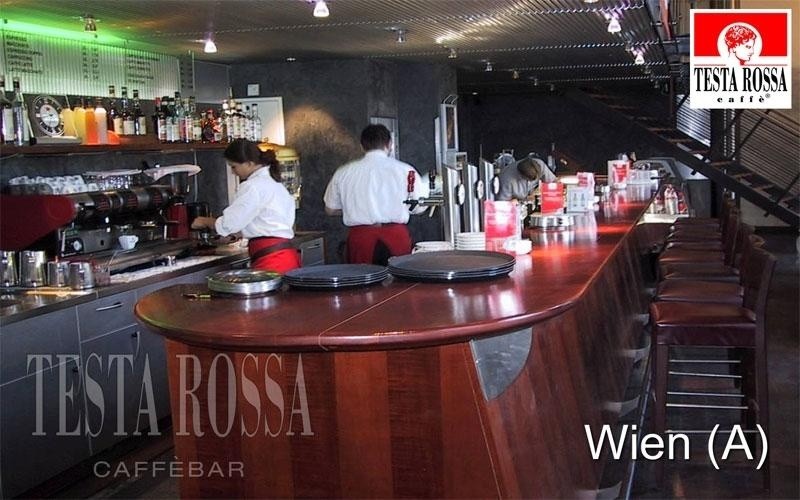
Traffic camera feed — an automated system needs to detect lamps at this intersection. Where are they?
[203,38,218,54]
[313,0,330,19]
[395,0,645,92]
[83,16,97,32]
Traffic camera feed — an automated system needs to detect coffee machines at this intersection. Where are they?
[185,201,211,240]
[53,186,186,259]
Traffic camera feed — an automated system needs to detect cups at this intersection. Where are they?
[119,235,138,250]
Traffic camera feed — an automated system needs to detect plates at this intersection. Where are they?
[285,262,388,289]
[207,270,283,295]
[388,251,516,280]
[455,233,486,250]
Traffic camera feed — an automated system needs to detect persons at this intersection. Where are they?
[189,137,302,278]
[322,124,430,270]
[494,156,558,228]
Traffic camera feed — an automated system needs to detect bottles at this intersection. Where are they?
[107,85,123,136]
[119,86,134,135]
[153,91,263,144]
[62,95,109,145]
[11,77,30,146]
[654,185,678,214]
[85,14,98,38]
[0,75,16,141]
[133,89,147,135]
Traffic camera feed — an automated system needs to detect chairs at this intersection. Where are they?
[649,190,780,491]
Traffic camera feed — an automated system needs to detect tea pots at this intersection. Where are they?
[49,260,70,286]
[71,262,96,291]
[0,251,19,286]
[20,250,49,287]
